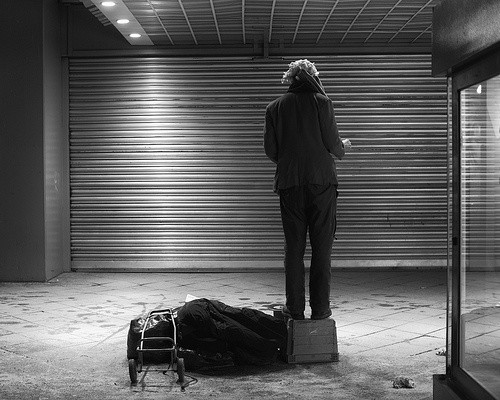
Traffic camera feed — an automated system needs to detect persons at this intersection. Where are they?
[262,58,352,320]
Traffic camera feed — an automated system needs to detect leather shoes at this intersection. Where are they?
[311,307,332,320]
[283,305,305,320]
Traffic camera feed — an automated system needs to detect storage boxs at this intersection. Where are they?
[273,307,340,365]
